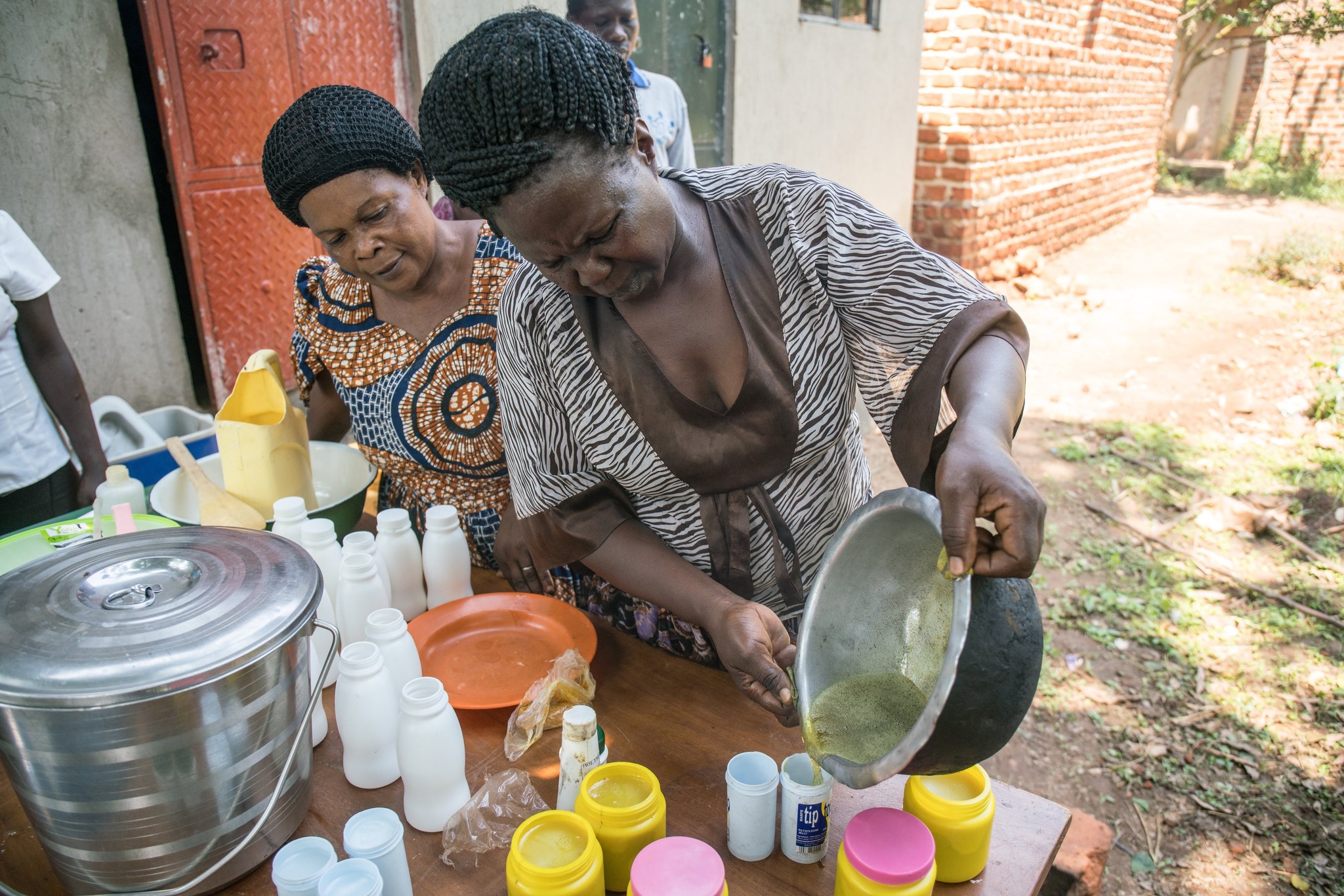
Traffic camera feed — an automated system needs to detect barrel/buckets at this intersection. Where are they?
[1,525,343,896]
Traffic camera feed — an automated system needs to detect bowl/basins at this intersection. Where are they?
[1,513,180,579]
[150,439,377,549]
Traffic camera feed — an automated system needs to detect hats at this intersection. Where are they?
[262,84,434,229]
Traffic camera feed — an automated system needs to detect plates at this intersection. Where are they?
[408,591,598,709]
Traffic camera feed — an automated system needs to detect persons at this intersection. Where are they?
[0,209,113,538]
[417,9,1050,731]
[562,0,697,171]
[432,194,485,221]
[260,81,720,673]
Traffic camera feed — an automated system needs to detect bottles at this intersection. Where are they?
[334,641,402,789]
[396,676,472,831]
[555,704,601,812]
[343,808,413,895]
[212,349,316,518]
[375,508,428,621]
[364,608,422,688]
[596,725,608,763]
[506,809,605,895]
[626,836,728,896]
[318,857,384,896]
[93,465,146,538]
[271,836,338,896]
[573,762,667,892]
[780,753,833,864]
[834,807,938,896]
[263,496,392,746]
[422,504,475,610]
[903,762,995,883]
[724,751,780,862]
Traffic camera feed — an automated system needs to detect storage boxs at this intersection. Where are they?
[107,404,217,486]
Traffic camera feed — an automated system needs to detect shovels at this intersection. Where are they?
[168,436,265,530]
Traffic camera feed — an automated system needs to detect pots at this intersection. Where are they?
[793,489,1043,789]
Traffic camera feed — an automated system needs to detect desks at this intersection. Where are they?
[0,504,1075,896]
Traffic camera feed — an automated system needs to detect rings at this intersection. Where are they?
[522,566,533,570]
